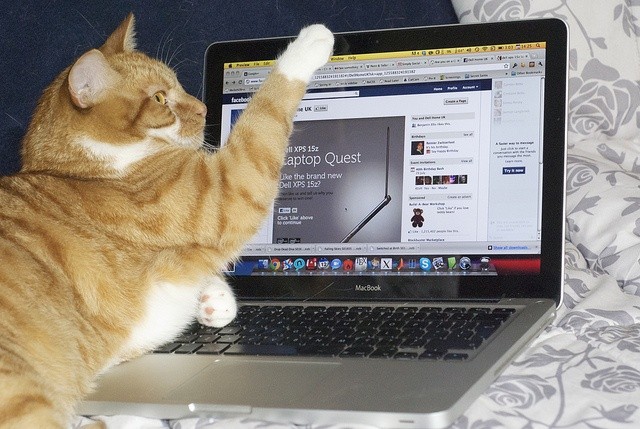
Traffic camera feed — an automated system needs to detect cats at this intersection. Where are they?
[0,10,335,429]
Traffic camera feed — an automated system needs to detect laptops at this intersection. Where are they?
[73,17,571,429]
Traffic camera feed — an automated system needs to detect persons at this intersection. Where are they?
[414,141,424,154]
